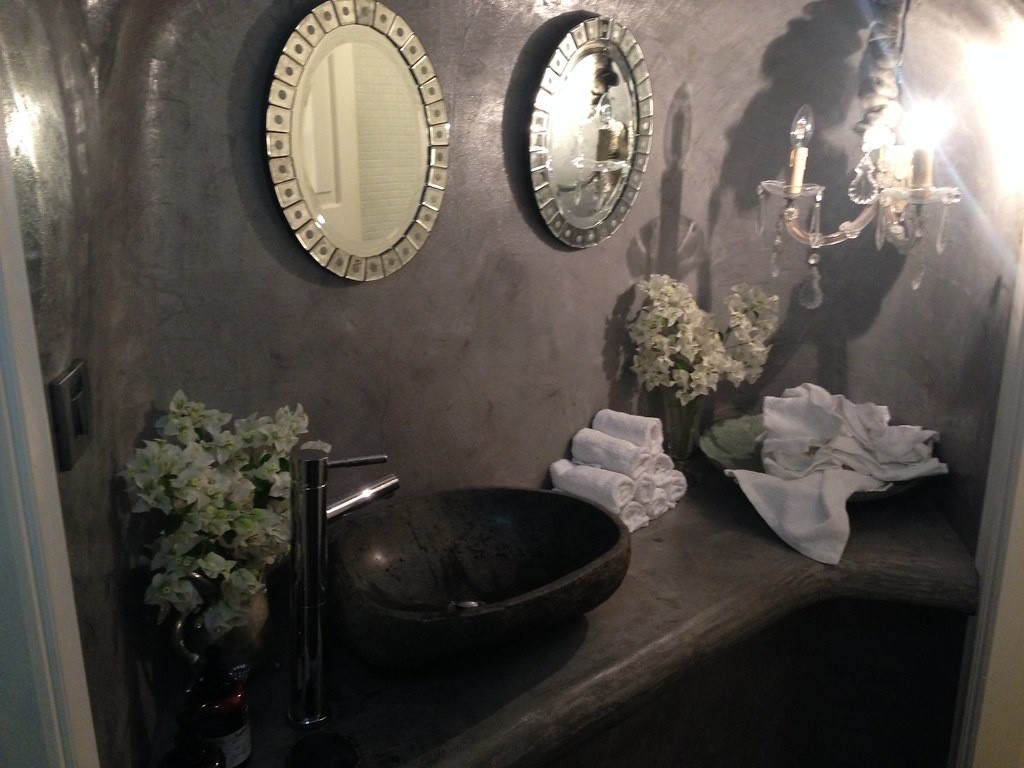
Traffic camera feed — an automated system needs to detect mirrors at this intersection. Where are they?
[529,18,654,249]
[265,1,448,280]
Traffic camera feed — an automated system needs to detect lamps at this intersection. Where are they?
[755,102,960,311]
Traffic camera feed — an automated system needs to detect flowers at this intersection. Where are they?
[114,389,332,639]
[625,276,776,407]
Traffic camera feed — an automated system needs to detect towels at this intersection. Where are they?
[723,381,949,564]
[553,409,688,536]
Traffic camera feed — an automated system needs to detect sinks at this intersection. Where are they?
[326,487,633,684]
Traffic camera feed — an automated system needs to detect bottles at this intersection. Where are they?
[186,644,252,768]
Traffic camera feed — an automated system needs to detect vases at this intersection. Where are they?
[661,389,708,466]
[172,570,271,666]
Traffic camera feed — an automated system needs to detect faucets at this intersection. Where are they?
[291,437,400,736]
